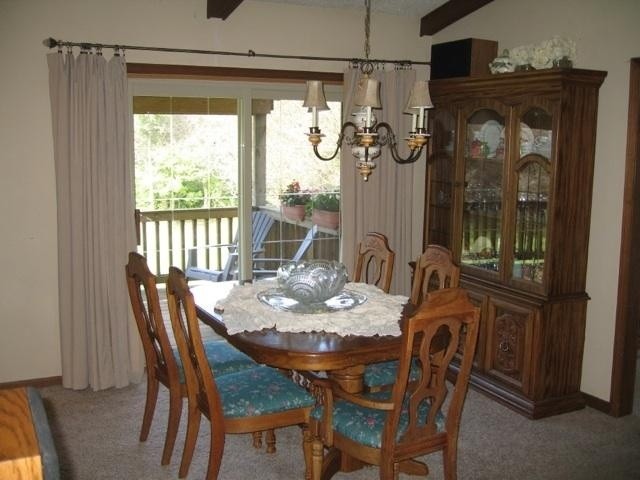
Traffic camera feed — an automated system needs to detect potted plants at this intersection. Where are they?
[278,179,340,229]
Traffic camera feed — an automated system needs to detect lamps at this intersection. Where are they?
[302,0,434,181]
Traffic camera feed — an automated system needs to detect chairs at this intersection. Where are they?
[165,266,316,477]
[303,289,480,477]
[126,252,262,465]
[352,234,461,411]
[185,211,318,282]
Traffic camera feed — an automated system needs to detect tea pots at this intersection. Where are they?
[487,48,517,74]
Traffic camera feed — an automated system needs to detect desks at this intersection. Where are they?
[188,280,452,472]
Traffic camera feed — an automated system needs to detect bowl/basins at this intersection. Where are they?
[276,260,347,304]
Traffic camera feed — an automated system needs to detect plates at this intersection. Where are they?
[256,287,368,315]
[477,119,535,160]
[511,35,576,70]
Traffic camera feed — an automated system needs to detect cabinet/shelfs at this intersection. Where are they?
[416,68,608,419]
